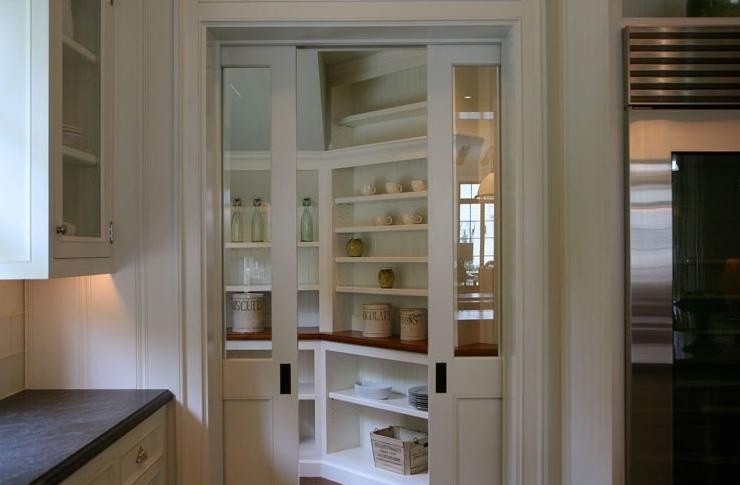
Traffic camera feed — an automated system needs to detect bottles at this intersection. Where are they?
[231,198,245,242]
[251,198,265,243]
[300,199,314,242]
[346,237,364,256]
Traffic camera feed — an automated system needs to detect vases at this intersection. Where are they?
[347,239,363,257]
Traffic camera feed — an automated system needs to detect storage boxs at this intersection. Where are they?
[370,426,428,475]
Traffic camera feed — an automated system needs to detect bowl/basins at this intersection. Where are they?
[354,380,392,400]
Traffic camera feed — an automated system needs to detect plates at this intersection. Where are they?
[408,385,428,412]
[62,122,87,144]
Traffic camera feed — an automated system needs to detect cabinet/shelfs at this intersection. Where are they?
[218,40,506,485]
[0,0,116,279]
[61,404,177,485]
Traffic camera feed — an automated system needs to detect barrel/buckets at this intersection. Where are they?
[232,293,265,333]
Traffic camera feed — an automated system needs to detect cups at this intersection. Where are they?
[385,182,402,193]
[374,215,393,225]
[402,214,422,224]
[361,184,375,194]
[411,180,425,191]
